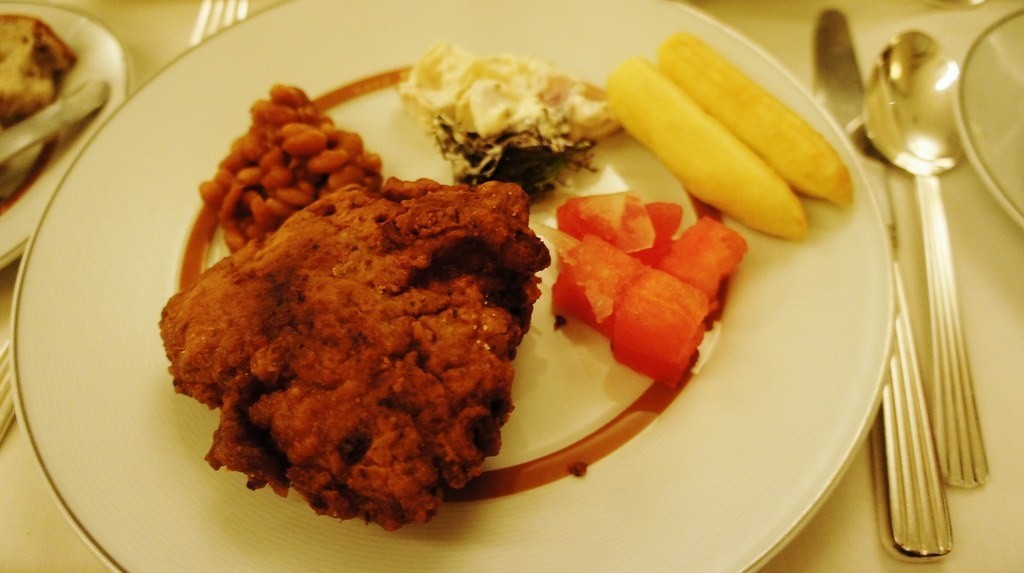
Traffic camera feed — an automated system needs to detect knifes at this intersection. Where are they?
[812,8,956,562]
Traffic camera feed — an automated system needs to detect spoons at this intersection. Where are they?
[863,29,996,489]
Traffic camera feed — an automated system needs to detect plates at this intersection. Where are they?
[957,2,1024,233]
[10,0,896,571]
[0,2,128,269]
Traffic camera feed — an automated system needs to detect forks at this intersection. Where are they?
[185,2,247,49]
